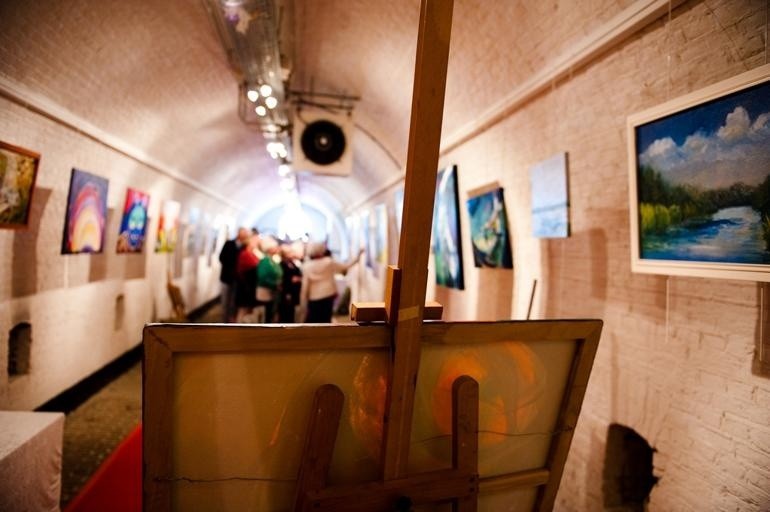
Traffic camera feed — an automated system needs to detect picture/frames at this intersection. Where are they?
[625,64,770,282]
[0,140,42,230]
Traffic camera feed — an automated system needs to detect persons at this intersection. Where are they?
[217,225,367,324]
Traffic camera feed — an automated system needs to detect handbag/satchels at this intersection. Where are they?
[255,284,274,302]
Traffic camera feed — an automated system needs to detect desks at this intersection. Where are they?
[0,410,66,512]
